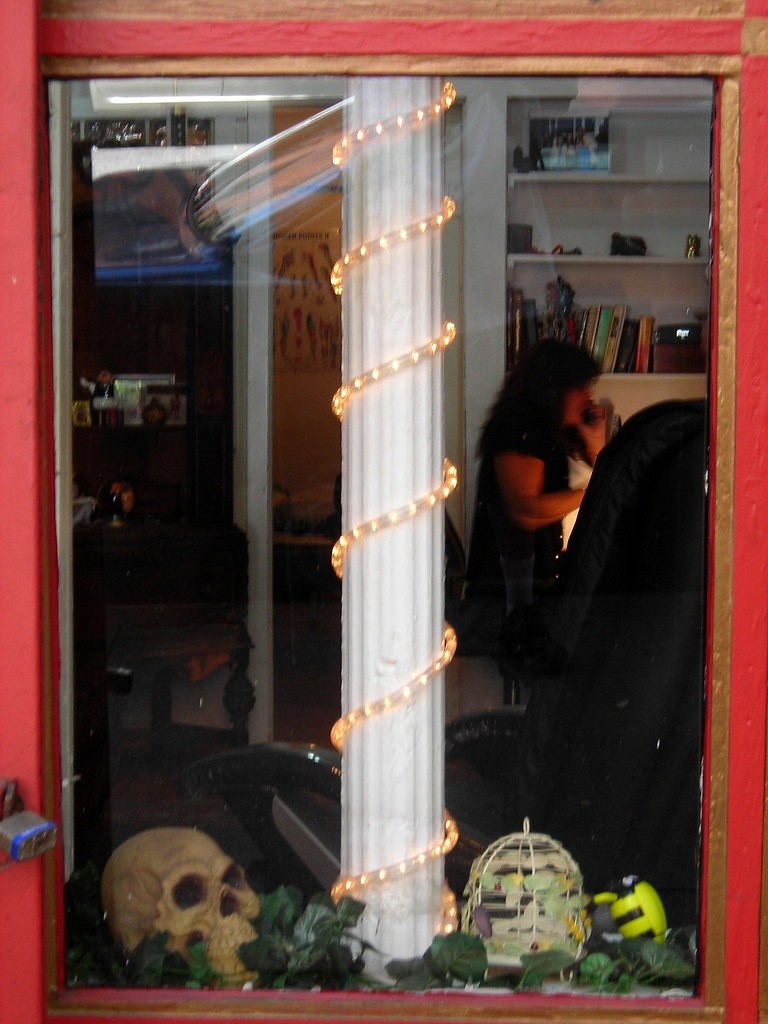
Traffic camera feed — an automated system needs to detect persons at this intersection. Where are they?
[167,395,181,421]
[462,338,604,663]
[140,398,167,428]
[531,127,585,171]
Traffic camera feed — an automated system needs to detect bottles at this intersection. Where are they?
[72,376,92,429]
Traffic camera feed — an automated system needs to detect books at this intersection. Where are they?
[574,304,656,373]
[506,284,537,383]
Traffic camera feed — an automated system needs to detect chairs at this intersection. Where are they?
[176,453,710,925]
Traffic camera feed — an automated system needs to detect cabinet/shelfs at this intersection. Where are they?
[71,118,272,605]
[493,96,714,379]
